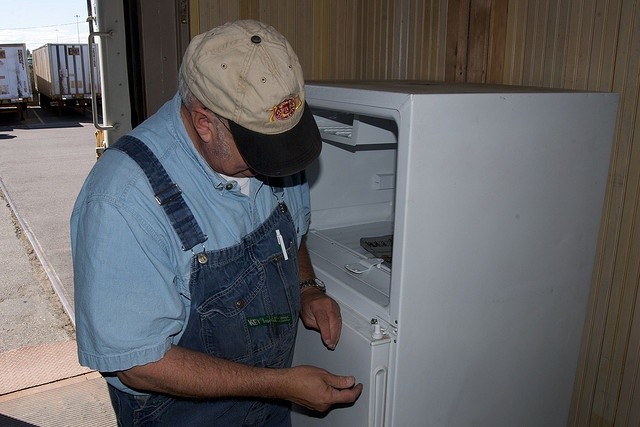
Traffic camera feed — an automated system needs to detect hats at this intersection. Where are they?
[181,21,322,177]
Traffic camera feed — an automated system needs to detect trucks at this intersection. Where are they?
[31,43,100,113]
[1,43,35,119]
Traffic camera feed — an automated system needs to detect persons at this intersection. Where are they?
[70,17,363,426]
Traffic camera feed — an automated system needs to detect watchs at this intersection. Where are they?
[299,277,326,290]
